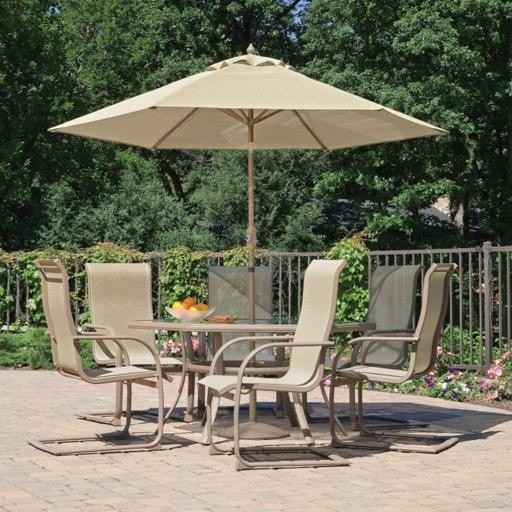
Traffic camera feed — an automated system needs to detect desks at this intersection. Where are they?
[126,318,377,441]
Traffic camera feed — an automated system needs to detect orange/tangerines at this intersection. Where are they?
[183,298,195,309]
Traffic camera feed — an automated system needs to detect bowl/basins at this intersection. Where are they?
[164,305,216,323]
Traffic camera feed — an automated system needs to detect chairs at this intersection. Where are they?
[199,265,285,422]
[197,259,346,473]
[28,258,173,457]
[302,264,430,431]
[328,261,460,455]
[74,261,195,427]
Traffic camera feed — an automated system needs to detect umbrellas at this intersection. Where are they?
[47,43,455,422]
